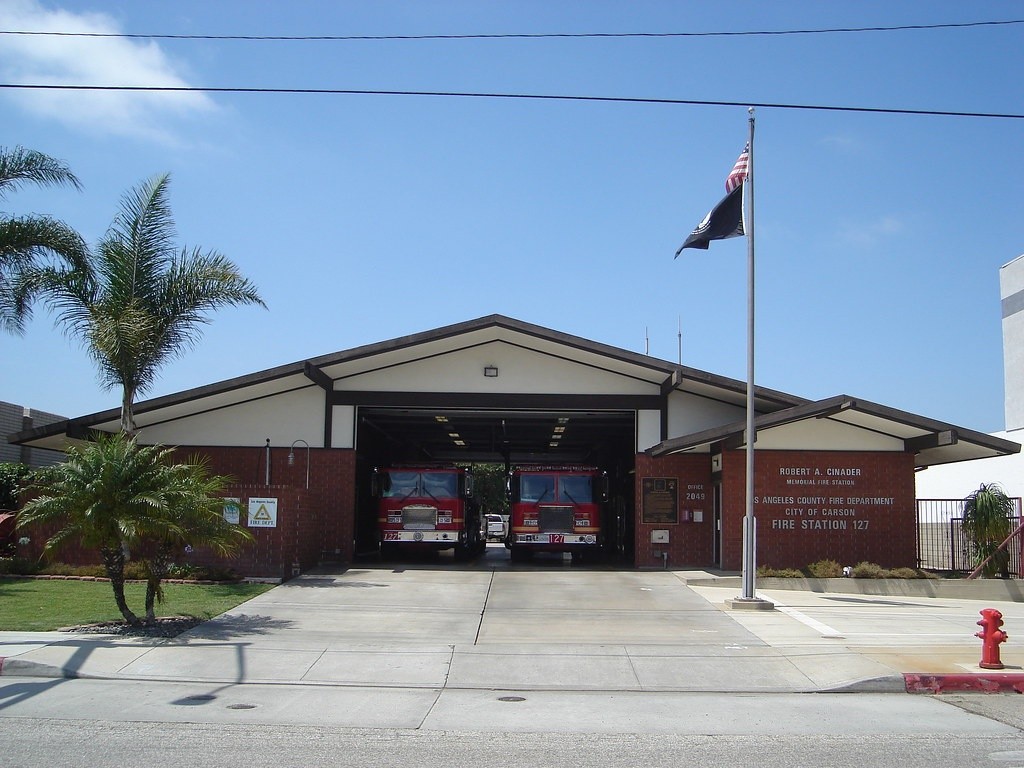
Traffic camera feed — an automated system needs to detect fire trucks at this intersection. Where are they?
[504,459,610,565]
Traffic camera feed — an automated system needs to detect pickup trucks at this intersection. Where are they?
[482,513,506,543]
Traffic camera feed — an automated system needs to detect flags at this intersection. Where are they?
[724,116,755,194]
[674,183,747,260]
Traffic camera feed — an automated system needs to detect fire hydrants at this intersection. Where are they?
[974,609,1009,669]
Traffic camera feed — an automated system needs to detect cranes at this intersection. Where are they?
[371,455,482,562]
